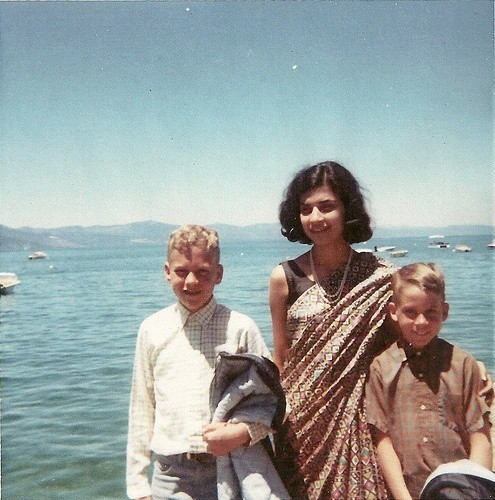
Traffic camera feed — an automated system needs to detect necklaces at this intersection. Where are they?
[309,246,354,303]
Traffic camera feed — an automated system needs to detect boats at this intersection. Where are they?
[28,252,47,260]
[428,235,450,248]
[455,244,471,252]
[487,240,495,248]
[375,246,395,251]
[0,272,20,294]
[355,249,373,253]
[389,250,408,257]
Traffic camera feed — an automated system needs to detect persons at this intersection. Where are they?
[268,162,493,500]
[365,262,495,500]
[125,226,275,500]
